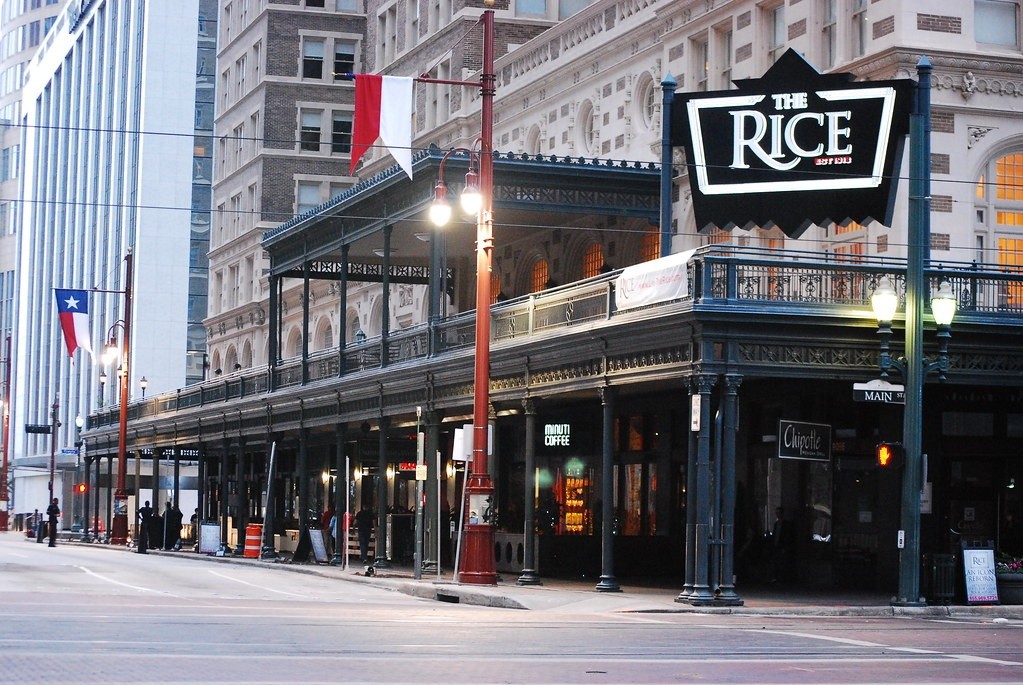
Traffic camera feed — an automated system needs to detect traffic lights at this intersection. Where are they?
[76,482,88,494]
[876,442,904,470]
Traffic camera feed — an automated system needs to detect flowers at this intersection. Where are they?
[995,553,1023,576]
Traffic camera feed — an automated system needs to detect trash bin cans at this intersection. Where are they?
[39,521,50,539]
[921,552,956,606]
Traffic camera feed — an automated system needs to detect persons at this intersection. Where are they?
[24,509,41,537]
[190,508,198,547]
[326,508,336,555]
[161,501,175,548]
[322,503,336,555]
[353,502,376,564]
[771,506,796,586]
[136,501,156,550]
[47,498,60,540]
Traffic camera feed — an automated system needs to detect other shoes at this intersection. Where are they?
[49,544,55,547]
[362,560,366,564]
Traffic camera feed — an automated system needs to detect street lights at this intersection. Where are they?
[866,115,958,609]
[117,365,123,407]
[0,328,14,532]
[70,412,83,532]
[429,10,501,585]
[139,375,148,401]
[99,371,108,407]
[99,247,135,545]
[45,401,63,505]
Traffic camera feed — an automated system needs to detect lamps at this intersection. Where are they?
[356,329,366,346]
[232,364,240,369]
[214,368,222,375]
[322,471,328,483]
[387,463,392,480]
[355,466,360,480]
[446,459,453,477]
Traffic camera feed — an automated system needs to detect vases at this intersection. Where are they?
[997,573,1023,604]
